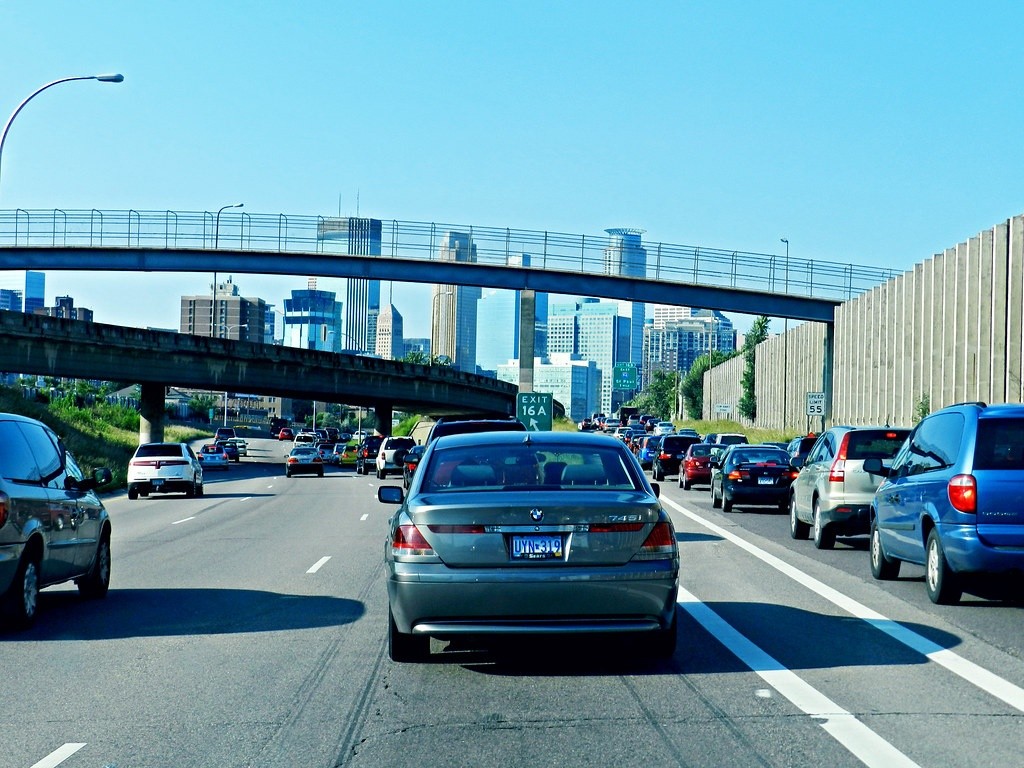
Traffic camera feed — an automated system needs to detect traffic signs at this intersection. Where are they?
[516,393,553,431]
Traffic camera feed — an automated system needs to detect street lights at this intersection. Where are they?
[212,204,250,339]
[327,332,365,353]
[780,238,789,294]
[1,73,125,174]
[430,291,455,366]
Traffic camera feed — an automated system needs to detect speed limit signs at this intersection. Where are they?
[806,392,825,416]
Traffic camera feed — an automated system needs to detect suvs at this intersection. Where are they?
[787,425,914,550]
[0,412,111,627]
[869,401,1024,605]
[126,442,204,499]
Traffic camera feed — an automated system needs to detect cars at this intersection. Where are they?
[582,408,820,516]
[378,430,680,664]
[197,427,249,467]
[270,418,530,491]
